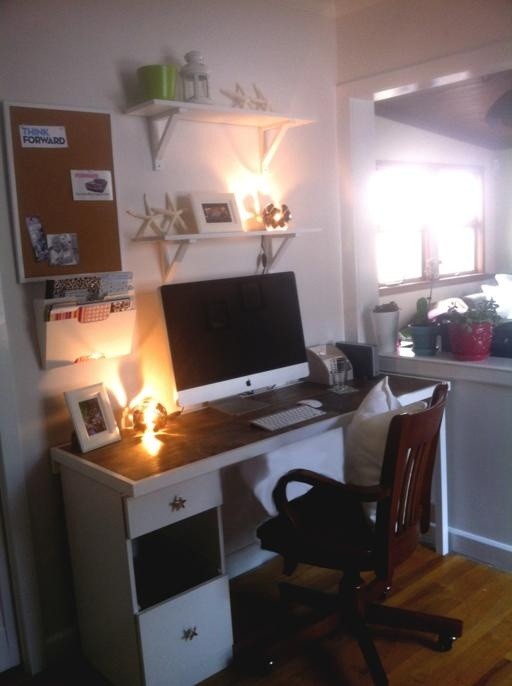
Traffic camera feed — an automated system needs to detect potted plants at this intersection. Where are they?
[406,300,438,354]
[447,302,499,361]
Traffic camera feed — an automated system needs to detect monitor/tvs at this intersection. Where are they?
[158,270,309,417]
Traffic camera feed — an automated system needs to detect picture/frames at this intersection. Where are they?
[62,383,121,453]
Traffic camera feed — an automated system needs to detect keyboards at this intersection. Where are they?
[251,405,325,432]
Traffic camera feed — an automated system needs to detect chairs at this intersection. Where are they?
[230,384,463,684]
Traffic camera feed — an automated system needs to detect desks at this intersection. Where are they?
[47,373,453,685]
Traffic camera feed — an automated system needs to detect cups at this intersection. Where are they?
[137,63,178,100]
[330,358,347,392]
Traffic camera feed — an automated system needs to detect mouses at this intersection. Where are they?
[297,398,323,410]
